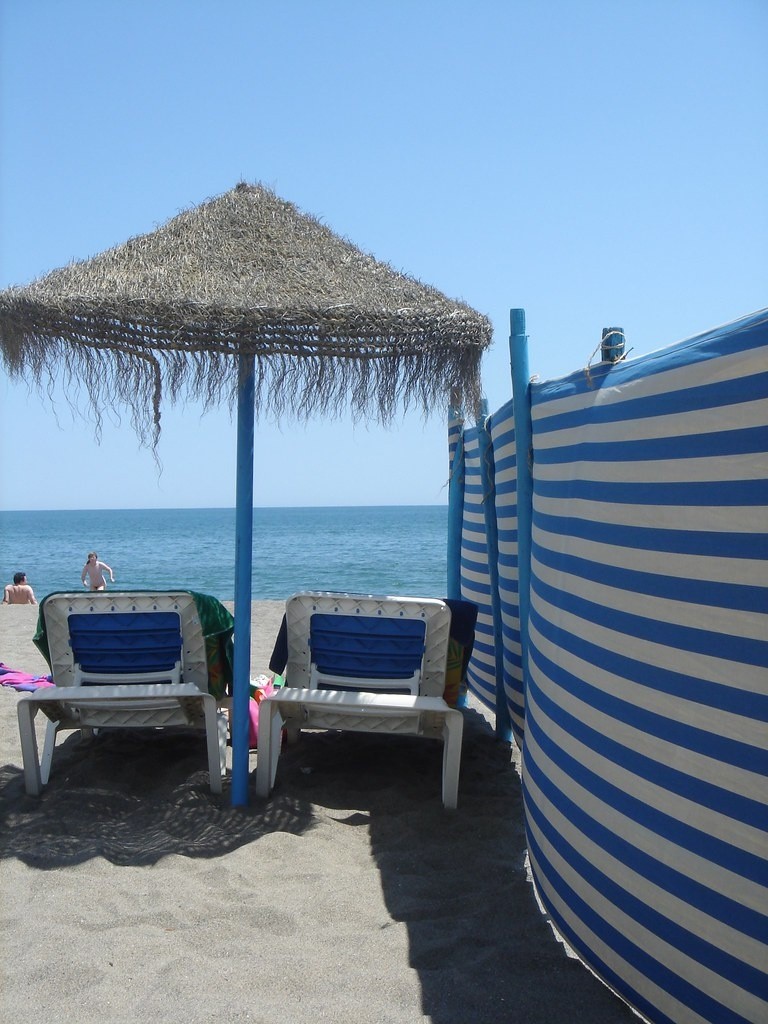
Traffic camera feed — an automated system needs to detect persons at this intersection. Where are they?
[80,552,115,591]
[3,573,38,606]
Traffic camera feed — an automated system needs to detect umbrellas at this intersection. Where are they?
[0,182,493,807]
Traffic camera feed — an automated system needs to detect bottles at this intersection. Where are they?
[273,674,284,689]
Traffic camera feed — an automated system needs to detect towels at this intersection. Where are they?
[0,663,285,752]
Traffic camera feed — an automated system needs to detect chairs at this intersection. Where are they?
[0,588,234,795]
[255,591,478,808]
